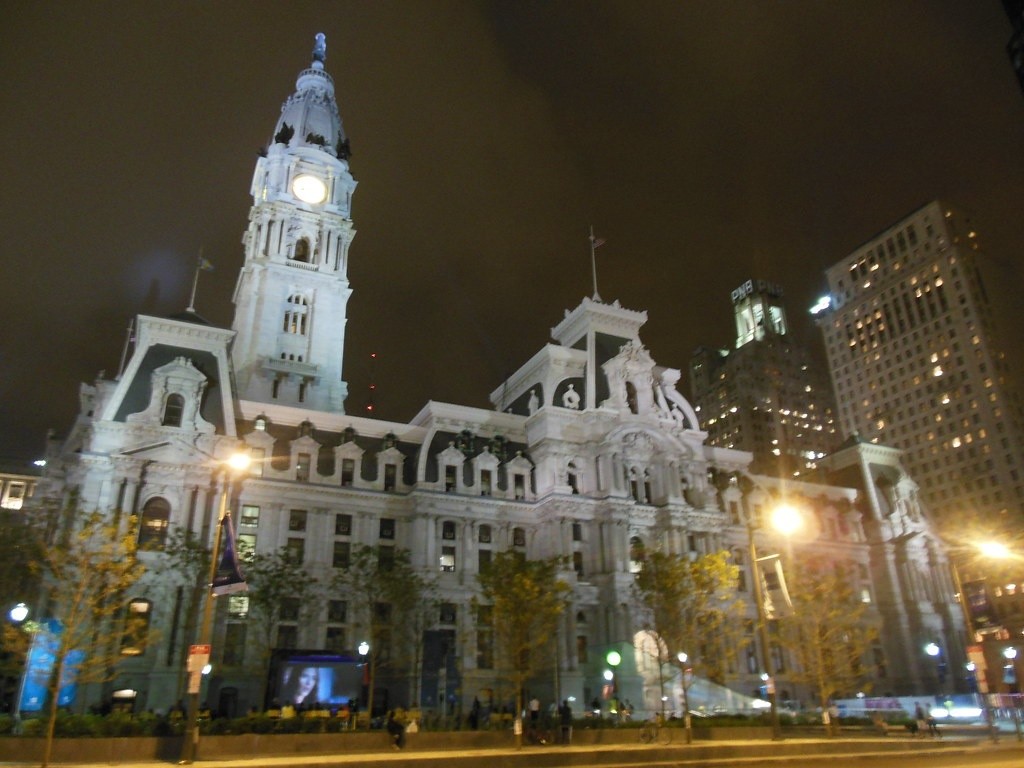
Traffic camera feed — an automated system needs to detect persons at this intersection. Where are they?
[106,704,210,728]
[287,666,319,706]
[243,695,633,750]
[915,700,944,740]
[872,711,889,737]
[653,711,679,729]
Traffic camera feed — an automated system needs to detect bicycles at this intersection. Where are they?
[638,721,674,745]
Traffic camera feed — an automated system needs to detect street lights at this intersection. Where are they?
[182,452,253,768]
[740,493,805,736]
[948,540,1010,739]
